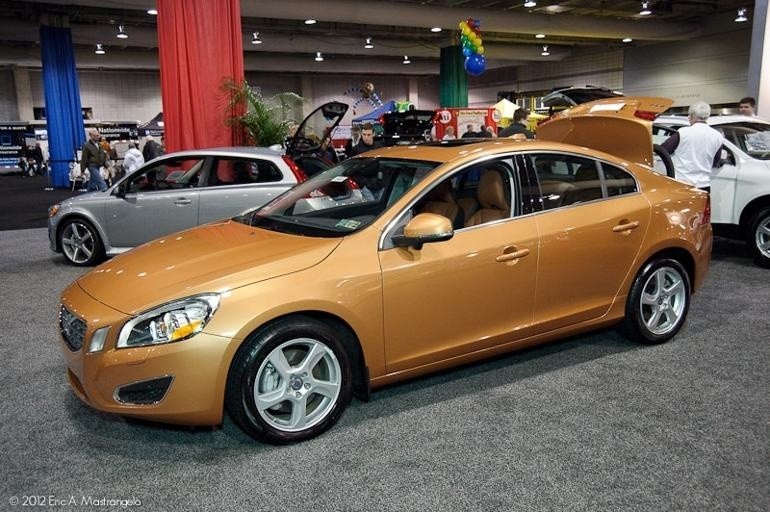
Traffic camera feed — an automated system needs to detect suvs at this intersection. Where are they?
[540,82,770,268]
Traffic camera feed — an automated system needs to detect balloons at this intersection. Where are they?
[459,18,488,77]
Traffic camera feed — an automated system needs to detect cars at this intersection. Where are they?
[55,97,714,445]
[48,102,350,269]
[20,106,438,181]
[716,125,770,161]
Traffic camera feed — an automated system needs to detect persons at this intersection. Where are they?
[461,107,536,138]
[343,125,362,160]
[739,97,770,153]
[19,143,43,176]
[81,127,165,192]
[659,101,723,193]
[442,126,456,140]
[313,136,339,165]
[350,123,386,157]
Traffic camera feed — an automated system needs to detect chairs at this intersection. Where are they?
[466,170,509,226]
[417,181,462,230]
